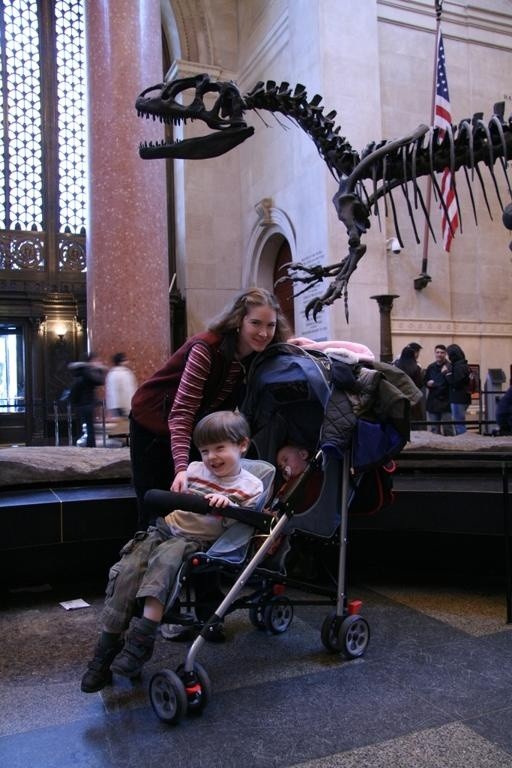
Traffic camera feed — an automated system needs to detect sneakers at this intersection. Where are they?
[109,621,157,676]
[79,636,125,694]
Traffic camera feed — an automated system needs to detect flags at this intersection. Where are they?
[434,25,460,253]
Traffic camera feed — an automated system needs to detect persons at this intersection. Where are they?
[79,411,264,694]
[388,343,512,436]
[64,352,109,447]
[105,351,139,416]
[255,442,321,559]
[118,288,290,643]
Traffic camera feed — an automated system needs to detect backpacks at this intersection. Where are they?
[452,364,479,395]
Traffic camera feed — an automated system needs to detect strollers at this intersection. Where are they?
[110,339,412,729]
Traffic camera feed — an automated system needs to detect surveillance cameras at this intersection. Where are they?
[386,237,400,254]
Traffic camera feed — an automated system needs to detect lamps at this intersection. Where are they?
[52,322,68,340]
